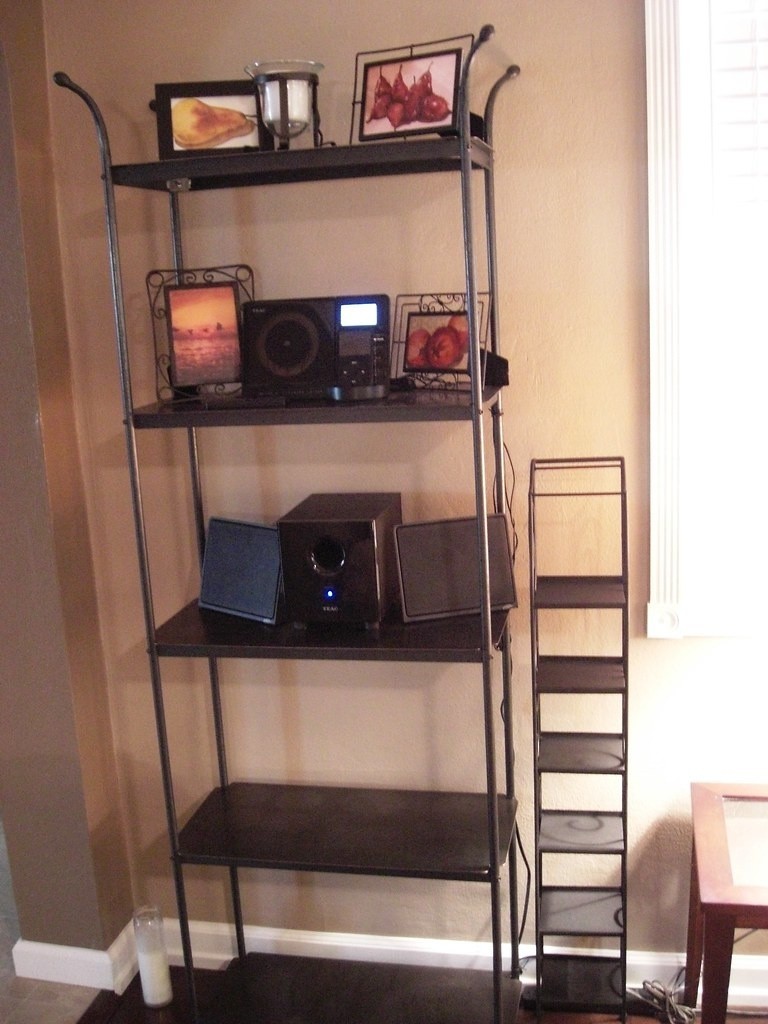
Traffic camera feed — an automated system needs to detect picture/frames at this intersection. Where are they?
[154,80,275,161]
[146,265,253,405]
[349,35,475,147]
[390,292,492,392]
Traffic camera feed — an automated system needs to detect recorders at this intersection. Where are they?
[238,293,391,402]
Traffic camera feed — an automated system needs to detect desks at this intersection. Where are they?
[684,783,768,1024]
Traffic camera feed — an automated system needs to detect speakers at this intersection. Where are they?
[394,512,519,623]
[197,517,283,625]
[276,492,404,630]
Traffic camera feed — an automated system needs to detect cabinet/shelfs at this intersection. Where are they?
[54,25,522,1024]
[528,456,628,1024]
